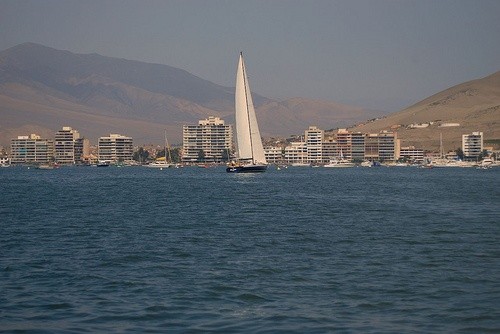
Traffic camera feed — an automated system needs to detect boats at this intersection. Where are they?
[36,162,60,170]
[87,132,228,169]
[281,156,500,169]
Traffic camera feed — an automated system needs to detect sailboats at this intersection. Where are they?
[225,52,268,172]
